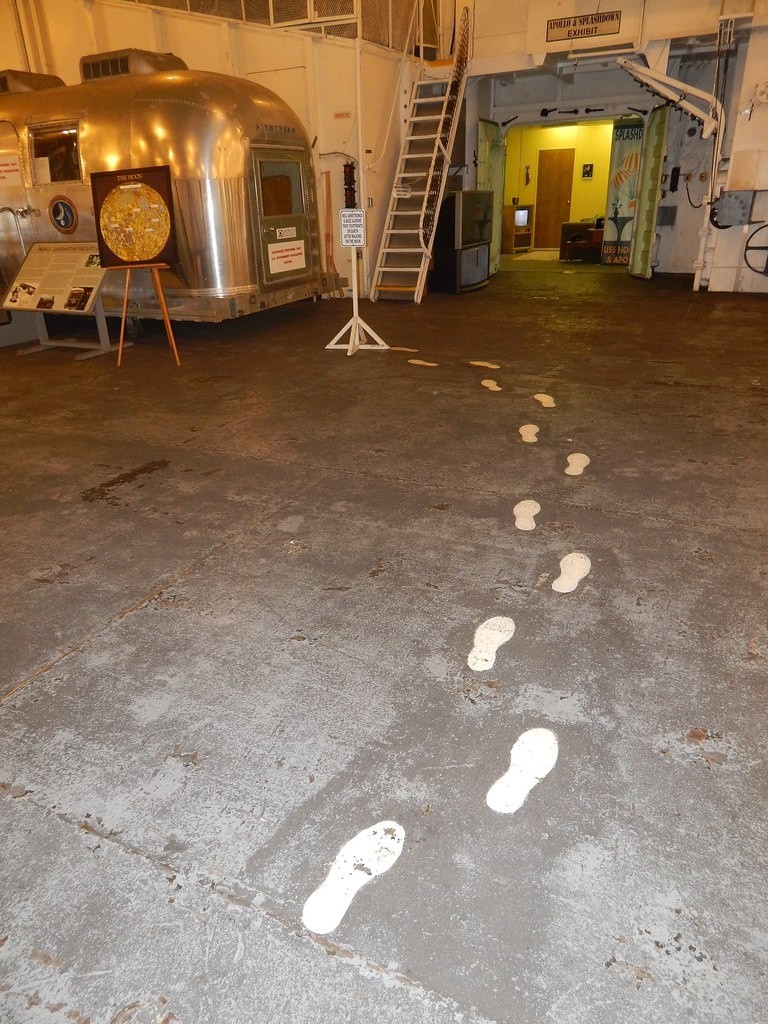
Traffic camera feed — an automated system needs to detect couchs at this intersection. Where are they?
[561,222,596,261]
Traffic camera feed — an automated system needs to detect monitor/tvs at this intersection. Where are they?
[516,208,530,227]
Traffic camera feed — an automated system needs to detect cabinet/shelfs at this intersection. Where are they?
[502,204,534,254]
[563,228,604,261]
